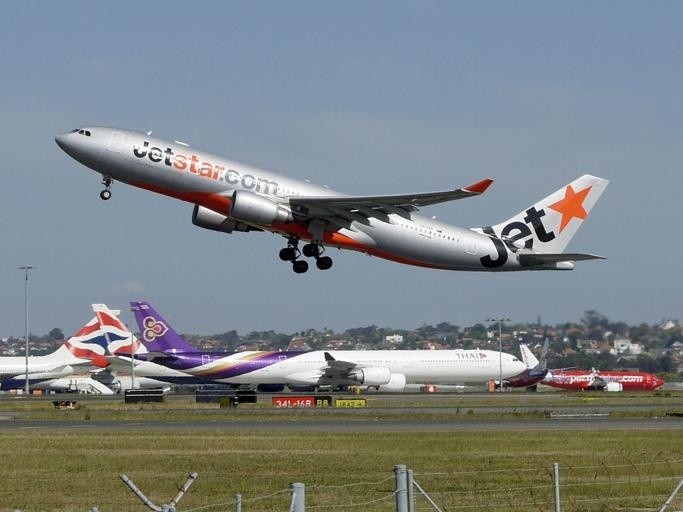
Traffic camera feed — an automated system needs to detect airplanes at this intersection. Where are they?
[51,112,618,283]
[516,339,668,399]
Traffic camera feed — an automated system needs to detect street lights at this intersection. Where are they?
[18,264,33,396]
